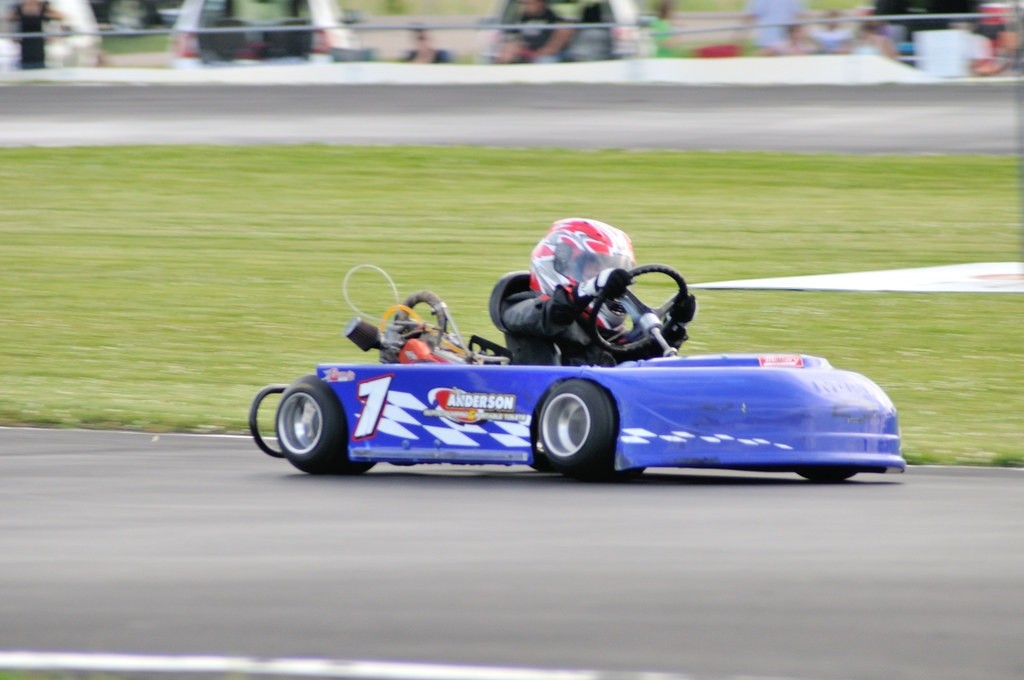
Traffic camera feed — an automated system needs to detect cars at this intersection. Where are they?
[0,1,105,68]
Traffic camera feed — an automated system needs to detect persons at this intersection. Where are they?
[404,30,449,63]
[742,0,1017,79]
[489,215,696,366]
[9,0,63,69]
[496,0,571,63]
[650,0,687,58]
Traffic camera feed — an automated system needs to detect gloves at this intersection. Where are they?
[665,294,696,324]
[574,269,633,302]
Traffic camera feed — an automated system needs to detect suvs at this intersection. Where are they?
[481,0,661,64]
[163,0,361,69]
[855,1,1024,77]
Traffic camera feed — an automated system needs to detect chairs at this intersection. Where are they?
[488,271,563,365]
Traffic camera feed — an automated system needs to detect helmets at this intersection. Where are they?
[530,216,633,298]
